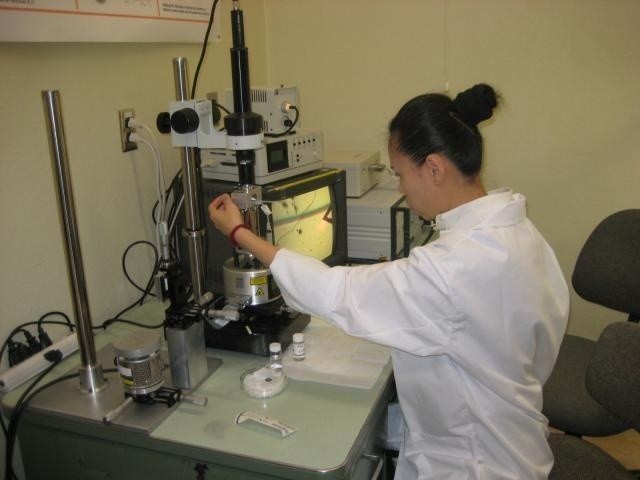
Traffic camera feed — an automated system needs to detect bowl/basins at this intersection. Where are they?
[239,368,288,398]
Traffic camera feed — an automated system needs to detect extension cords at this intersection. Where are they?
[0,331,79,393]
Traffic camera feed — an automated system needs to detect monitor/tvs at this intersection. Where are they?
[172,168,348,307]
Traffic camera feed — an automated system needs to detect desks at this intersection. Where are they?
[0,225,438,480]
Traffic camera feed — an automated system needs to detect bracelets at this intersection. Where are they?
[227,222,249,248]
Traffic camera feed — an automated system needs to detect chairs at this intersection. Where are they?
[548,322,640,480]
[539,209,640,437]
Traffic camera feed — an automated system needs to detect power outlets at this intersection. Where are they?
[117,107,139,152]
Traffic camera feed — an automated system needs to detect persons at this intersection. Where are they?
[208,83,570,479]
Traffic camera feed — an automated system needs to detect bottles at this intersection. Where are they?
[112,330,166,395]
[293,332,305,361]
[268,343,283,371]
